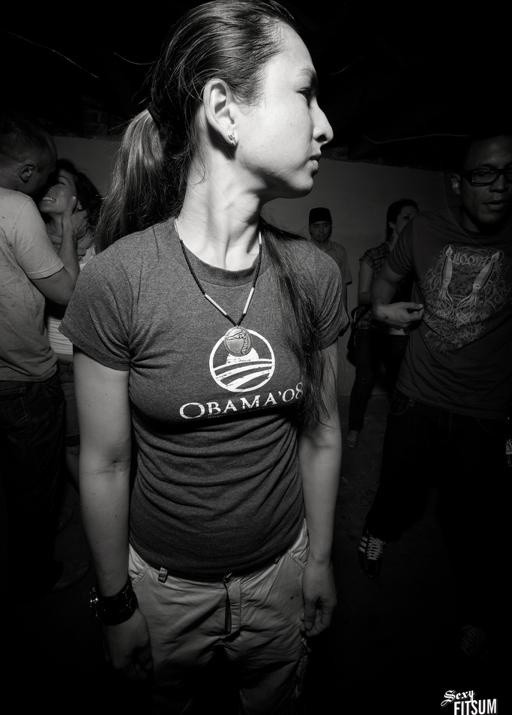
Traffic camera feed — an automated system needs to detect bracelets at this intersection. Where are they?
[87,574,139,625]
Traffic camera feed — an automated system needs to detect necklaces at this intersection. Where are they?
[175,219,262,357]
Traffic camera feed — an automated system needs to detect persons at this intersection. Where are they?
[35,158,104,494]
[0,129,91,593]
[308,207,352,337]
[357,123,512,679]
[57,12,349,715]
[346,199,420,450]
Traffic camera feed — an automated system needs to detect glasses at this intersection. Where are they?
[463,162,510,187]
